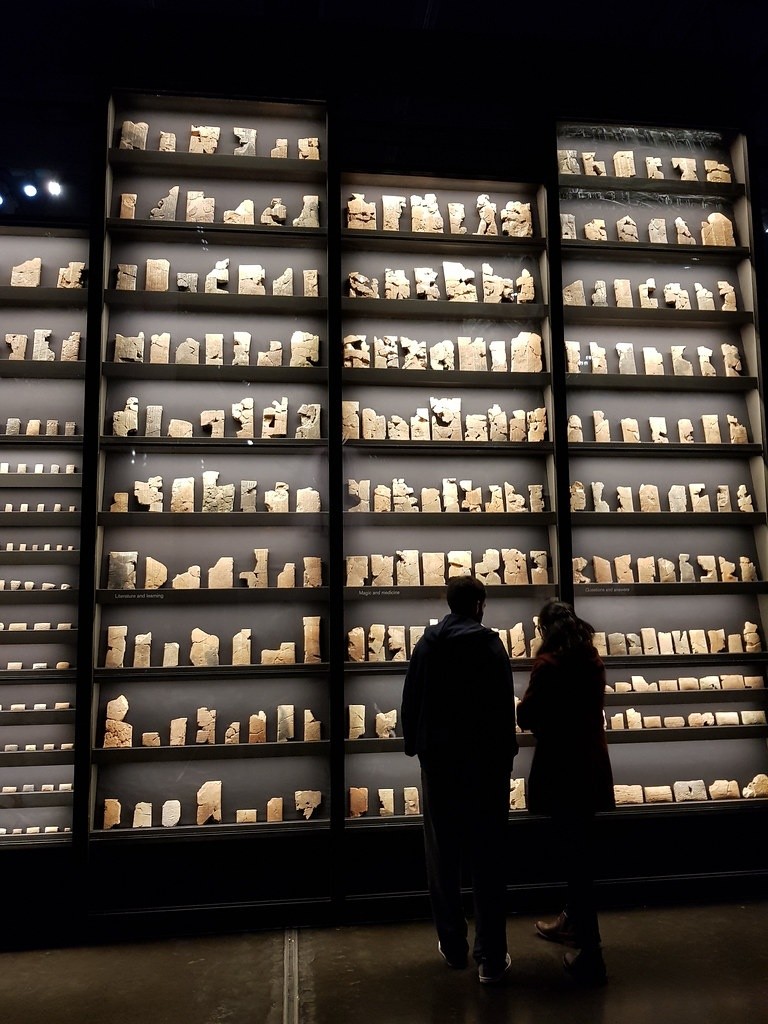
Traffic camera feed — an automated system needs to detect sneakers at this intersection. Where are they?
[478,952,511,983]
[437,941,467,968]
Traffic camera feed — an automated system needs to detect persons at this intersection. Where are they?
[516,601,616,985]
[401,576,519,982]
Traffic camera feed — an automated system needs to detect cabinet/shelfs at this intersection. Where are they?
[0,79,768,955]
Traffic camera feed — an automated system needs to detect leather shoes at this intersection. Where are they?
[534,911,576,946]
[562,946,606,985]
